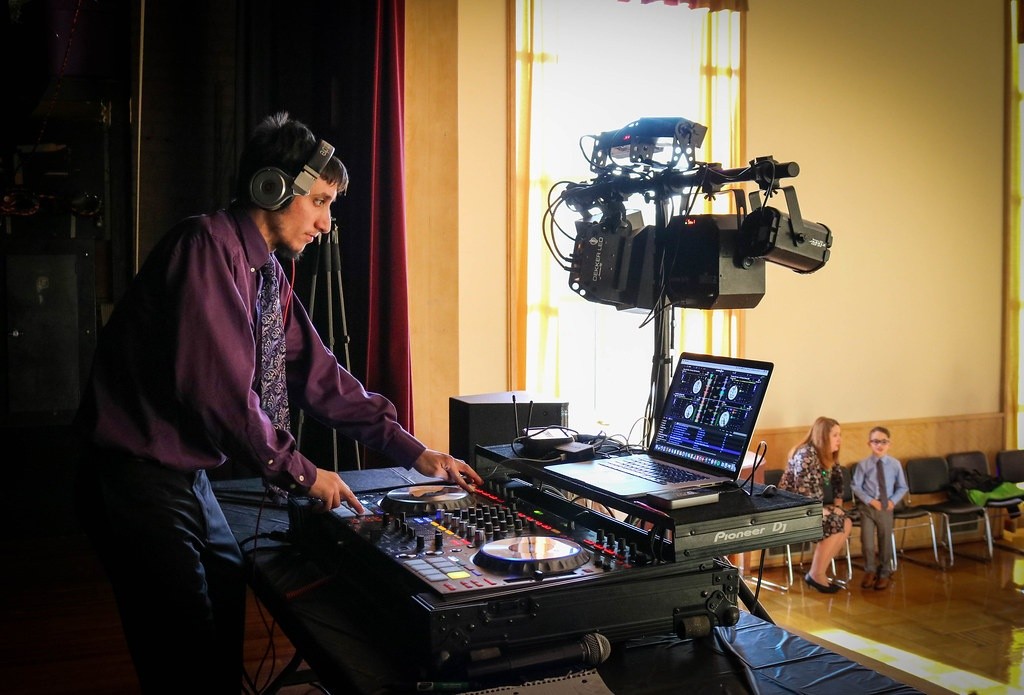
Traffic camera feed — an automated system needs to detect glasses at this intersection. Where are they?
[869,439,889,445]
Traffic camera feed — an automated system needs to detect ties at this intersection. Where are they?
[876,460,888,510]
[257,257,291,499]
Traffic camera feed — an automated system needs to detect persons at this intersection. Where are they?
[777,417,853,594]
[849,426,909,589]
[81,113,483,694]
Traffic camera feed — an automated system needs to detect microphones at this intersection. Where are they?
[465,633,611,680]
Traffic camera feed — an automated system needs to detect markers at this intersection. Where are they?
[381,680,481,691]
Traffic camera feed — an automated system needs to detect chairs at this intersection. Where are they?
[900,456,995,566]
[852,461,941,571]
[944,450,1024,550]
[995,448,1024,482]
[821,464,862,584]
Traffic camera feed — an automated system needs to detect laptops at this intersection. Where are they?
[543,350,775,497]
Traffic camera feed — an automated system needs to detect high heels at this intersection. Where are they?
[804,572,841,593]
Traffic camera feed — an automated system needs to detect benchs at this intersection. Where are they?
[748,469,837,592]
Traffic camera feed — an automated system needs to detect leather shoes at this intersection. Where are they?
[861,572,877,587]
[875,576,891,589]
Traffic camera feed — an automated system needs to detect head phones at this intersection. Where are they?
[249,138,336,212]
